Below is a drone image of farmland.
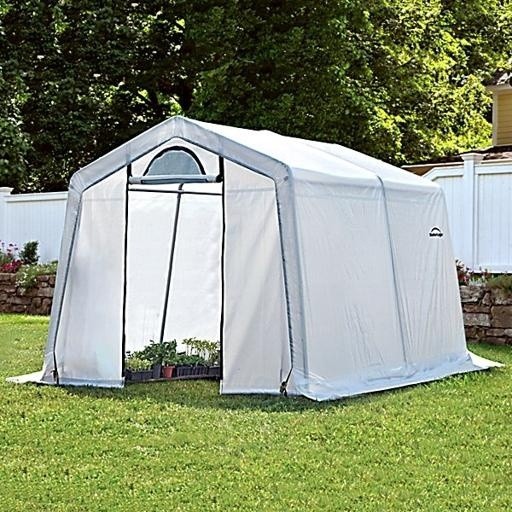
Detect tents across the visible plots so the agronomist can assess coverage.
[6,114,505,401]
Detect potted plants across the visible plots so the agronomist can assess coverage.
[121,339,224,383]
